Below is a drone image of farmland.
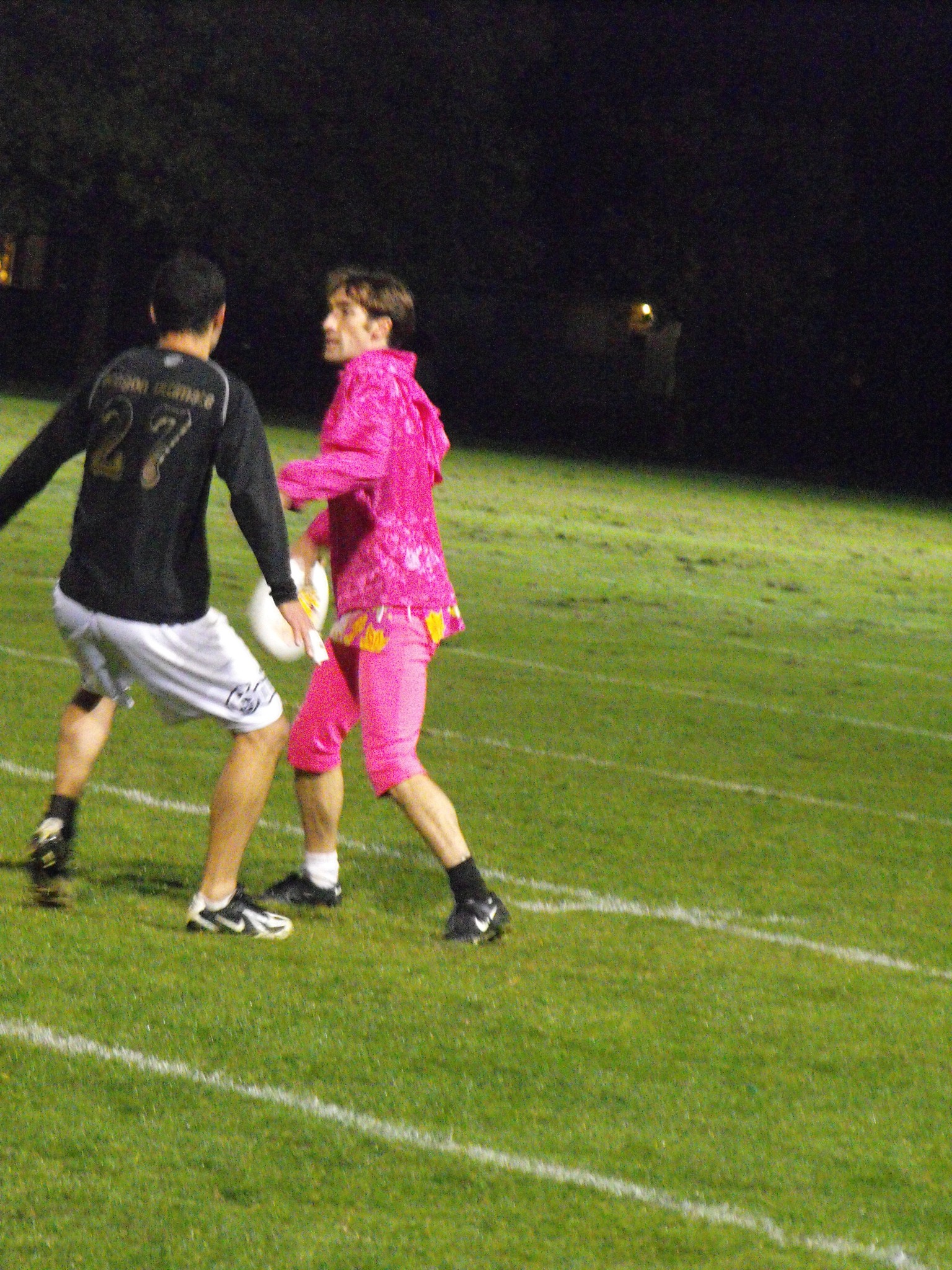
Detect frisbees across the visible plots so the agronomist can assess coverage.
[247,556,330,663]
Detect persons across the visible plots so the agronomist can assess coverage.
[250,269,511,946]
[0,254,329,941]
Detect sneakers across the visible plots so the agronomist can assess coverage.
[28,818,71,908]
[186,886,293,942]
[257,872,347,909]
[445,890,512,947]
